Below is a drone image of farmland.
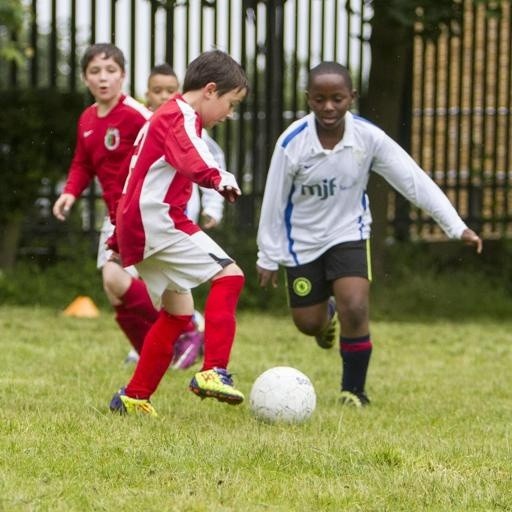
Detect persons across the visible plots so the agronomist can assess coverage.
[53,43,205,369]
[110,51,252,416]
[255,60,482,408]
[127,62,226,364]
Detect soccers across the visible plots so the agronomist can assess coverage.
[250,365,316,426]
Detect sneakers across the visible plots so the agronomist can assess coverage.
[316,295,338,349]
[109,385,161,420]
[337,390,369,408]
[188,366,245,406]
[169,309,206,371]
